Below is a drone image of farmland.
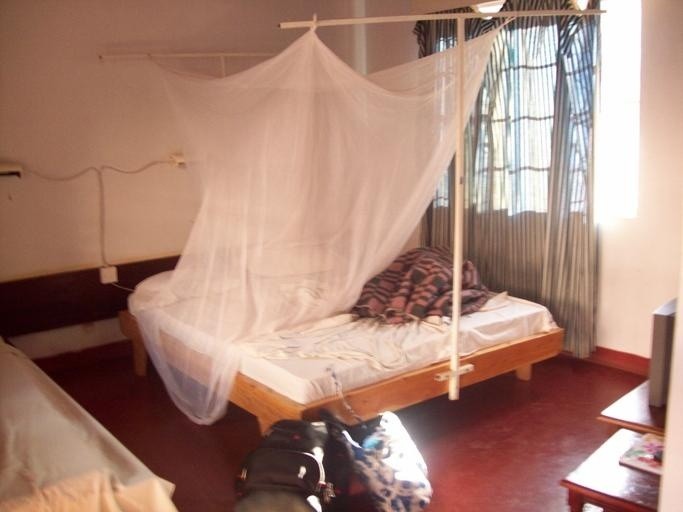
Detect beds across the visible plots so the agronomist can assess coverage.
[117,272,566,437]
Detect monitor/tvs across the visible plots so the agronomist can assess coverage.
[648,297,677,407]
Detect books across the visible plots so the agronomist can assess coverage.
[619,433,666,476]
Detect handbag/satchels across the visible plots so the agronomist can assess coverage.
[320,410,433,512]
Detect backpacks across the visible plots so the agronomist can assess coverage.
[233,419,349,512]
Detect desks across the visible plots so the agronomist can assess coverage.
[561,380,664,512]
